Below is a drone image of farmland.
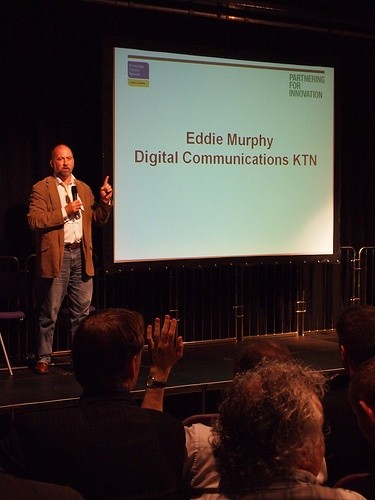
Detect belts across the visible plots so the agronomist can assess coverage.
[65,243,80,250]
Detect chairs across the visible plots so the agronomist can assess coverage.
[177,403,375,500]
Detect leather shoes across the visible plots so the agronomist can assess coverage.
[35,363,48,373]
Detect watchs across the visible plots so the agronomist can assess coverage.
[146,376,166,388]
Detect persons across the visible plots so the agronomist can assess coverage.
[28,144,112,375]
[317,305,374,500]
[187,360,365,500]
[2,308,185,500]
[141,314,327,489]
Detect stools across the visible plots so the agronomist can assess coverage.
[0,309,26,376]
[59,306,97,318]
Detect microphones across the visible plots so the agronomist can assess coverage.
[71,185,80,217]
